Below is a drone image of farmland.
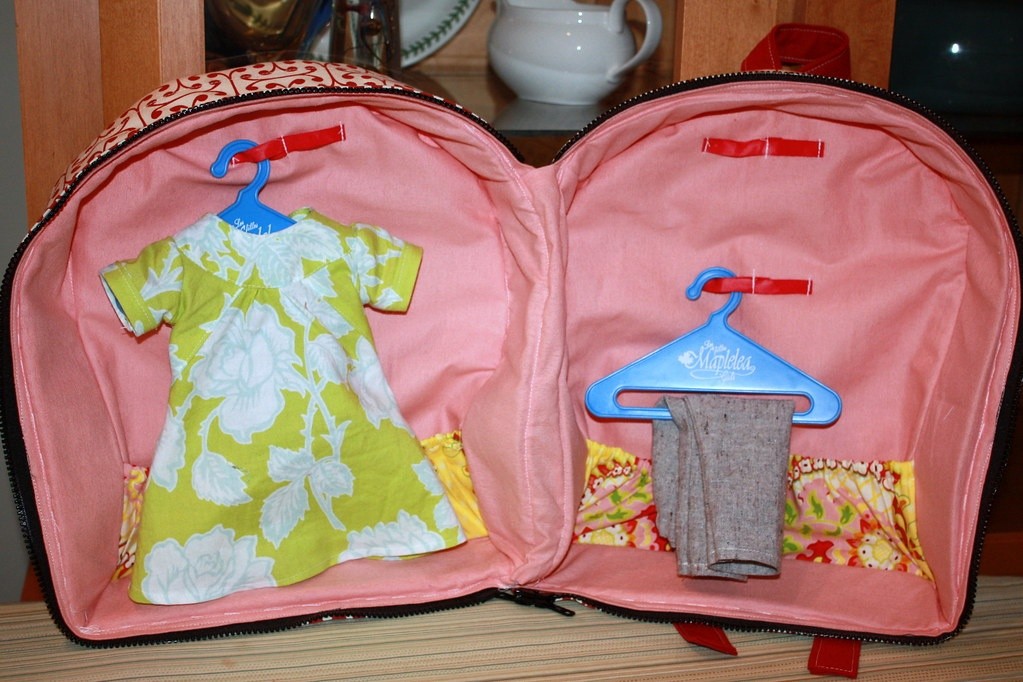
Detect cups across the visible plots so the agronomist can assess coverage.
[331,1,400,75]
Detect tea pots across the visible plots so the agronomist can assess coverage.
[485,2,665,107]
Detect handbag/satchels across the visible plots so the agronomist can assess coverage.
[1,62,1022,645]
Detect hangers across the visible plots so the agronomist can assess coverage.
[585,266,843,428]
[112,137,419,314]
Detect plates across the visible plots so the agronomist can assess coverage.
[307,1,478,69]
